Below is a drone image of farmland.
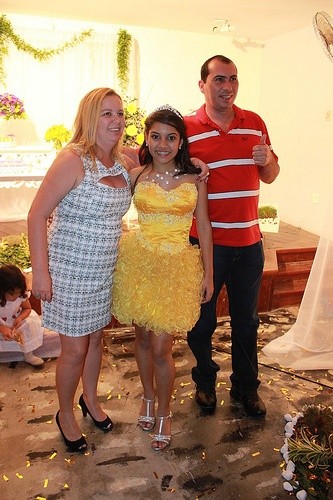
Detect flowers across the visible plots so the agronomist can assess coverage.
[119,94,148,151]
[0,89,29,127]
[1,234,31,269]
[43,122,74,151]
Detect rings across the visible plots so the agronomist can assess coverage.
[35,293,40,297]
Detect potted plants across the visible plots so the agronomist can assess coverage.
[256,204,281,235]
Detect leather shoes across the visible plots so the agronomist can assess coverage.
[229,384,266,416]
[194,383,216,409]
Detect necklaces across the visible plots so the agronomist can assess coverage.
[146,168,187,185]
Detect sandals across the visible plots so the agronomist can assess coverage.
[151,409,172,450]
[137,396,155,431]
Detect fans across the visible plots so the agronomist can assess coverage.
[311,9,333,65]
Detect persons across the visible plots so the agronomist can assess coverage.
[27,87,209,453]
[0,265,44,365]
[111,104,214,451]
[118,55,280,417]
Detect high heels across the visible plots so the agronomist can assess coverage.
[54,410,87,452]
[78,393,113,431]
